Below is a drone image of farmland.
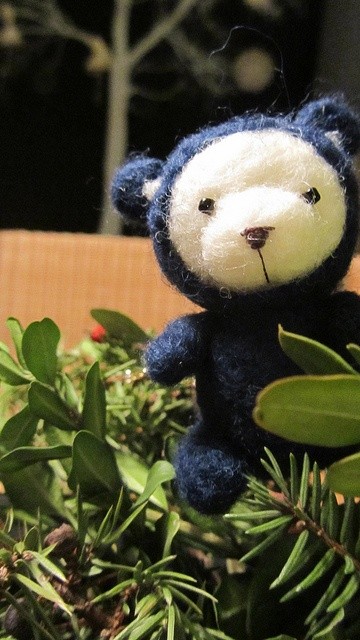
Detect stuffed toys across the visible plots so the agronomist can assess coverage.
[108,94,360,515]
